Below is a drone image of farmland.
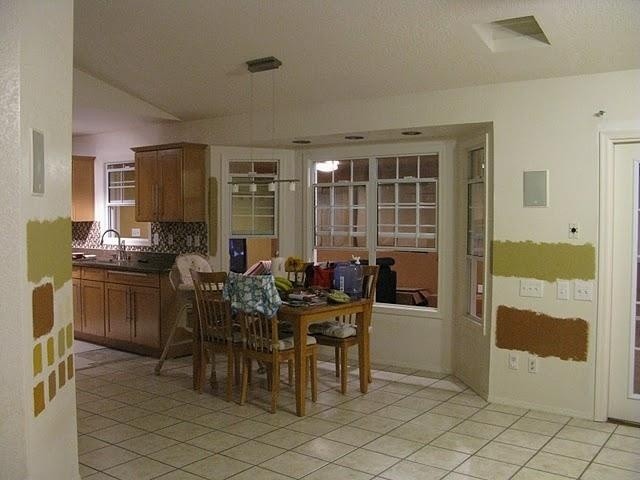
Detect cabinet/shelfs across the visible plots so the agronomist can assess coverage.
[129,142,208,224]
[72,265,161,358]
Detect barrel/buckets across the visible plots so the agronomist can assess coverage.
[333,261,364,301]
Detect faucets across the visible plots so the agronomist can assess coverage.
[100,229,125,260]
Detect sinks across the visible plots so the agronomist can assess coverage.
[97,259,116,265]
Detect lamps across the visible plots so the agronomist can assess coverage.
[227,56,300,193]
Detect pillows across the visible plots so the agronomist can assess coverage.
[241,260,273,275]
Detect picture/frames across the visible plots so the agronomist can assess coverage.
[30,127,46,196]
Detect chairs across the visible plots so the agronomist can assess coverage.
[153,251,381,414]
